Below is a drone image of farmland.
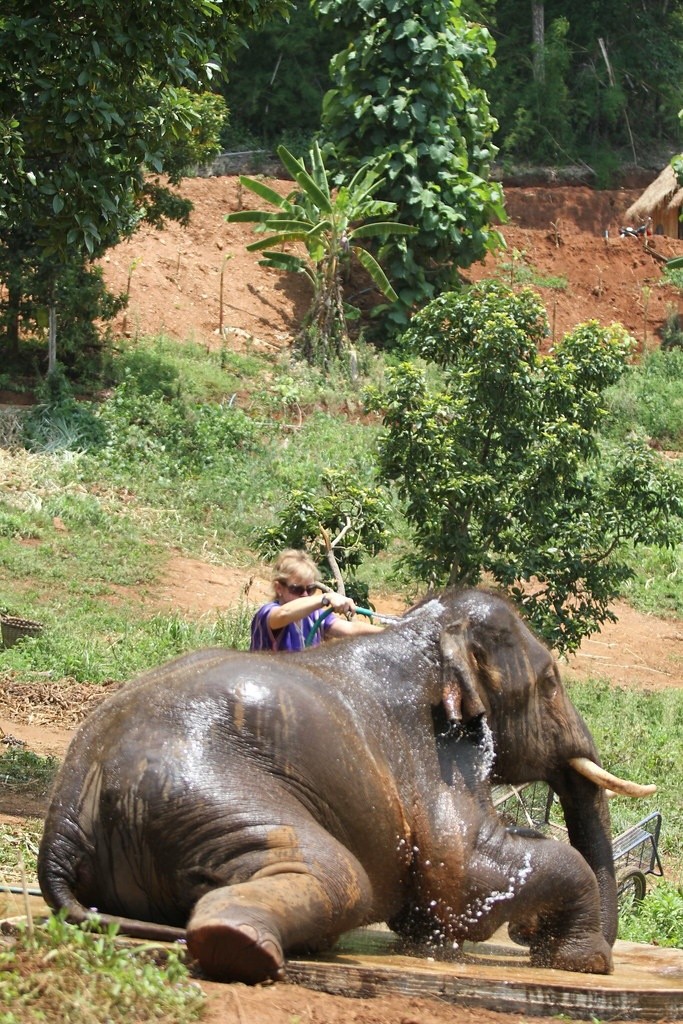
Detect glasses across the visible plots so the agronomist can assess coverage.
[277,579,316,596]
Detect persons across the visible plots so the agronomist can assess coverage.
[249,548,385,651]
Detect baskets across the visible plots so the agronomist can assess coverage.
[0,612,52,647]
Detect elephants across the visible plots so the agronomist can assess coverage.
[37,582,660,990]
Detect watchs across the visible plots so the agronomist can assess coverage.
[321,592,331,607]
